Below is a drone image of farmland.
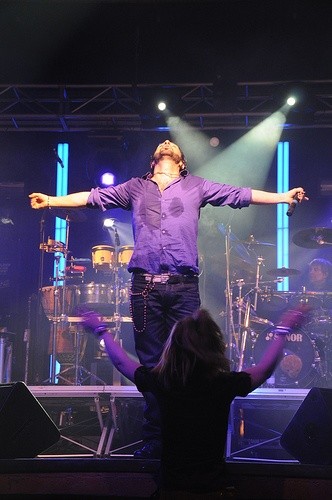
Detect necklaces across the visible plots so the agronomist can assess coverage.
[153,172,180,178]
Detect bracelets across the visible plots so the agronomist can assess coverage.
[46,196,51,209]
[271,325,294,335]
[94,324,108,335]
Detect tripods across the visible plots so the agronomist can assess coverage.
[43,324,105,386]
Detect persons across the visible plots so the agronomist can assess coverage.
[28,140,308,367]
[306,258,332,293]
[81,304,316,500]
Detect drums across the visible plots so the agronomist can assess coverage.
[306,308,332,327]
[39,285,74,354]
[90,245,115,269]
[117,246,134,267]
[54,325,88,386]
[76,283,114,308]
[252,325,324,388]
[119,279,131,307]
[231,269,272,312]
[248,294,287,328]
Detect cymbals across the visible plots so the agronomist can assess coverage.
[293,227,332,248]
[243,241,276,246]
[266,269,302,276]
[39,240,65,253]
[212,253,255,280]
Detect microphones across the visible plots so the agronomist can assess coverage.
[286,192,299,217]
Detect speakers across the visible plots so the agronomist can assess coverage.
[0,381,60,458]
[279,386,332,466]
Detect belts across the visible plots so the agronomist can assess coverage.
[132,274,198,285]
